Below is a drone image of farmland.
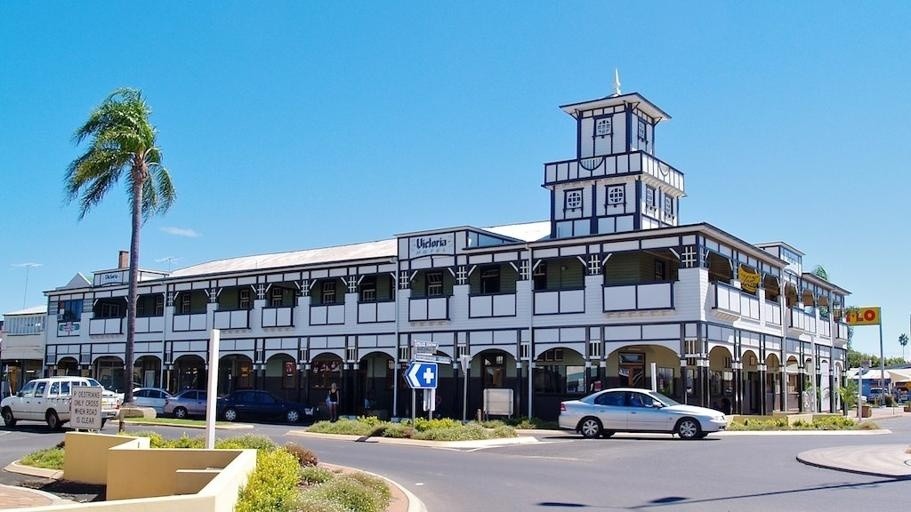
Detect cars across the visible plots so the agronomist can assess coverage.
[161,391,221,418]
[558,387,727,441]
[120,388,172,417]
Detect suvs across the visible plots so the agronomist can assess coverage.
[218,389,316,426]
[50,376,117,399]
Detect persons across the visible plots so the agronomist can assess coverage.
[326,389,331,407]
[327,382,339,423]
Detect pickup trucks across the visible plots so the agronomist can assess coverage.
[0,378,120,431]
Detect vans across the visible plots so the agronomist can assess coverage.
[867,387,888,402]
